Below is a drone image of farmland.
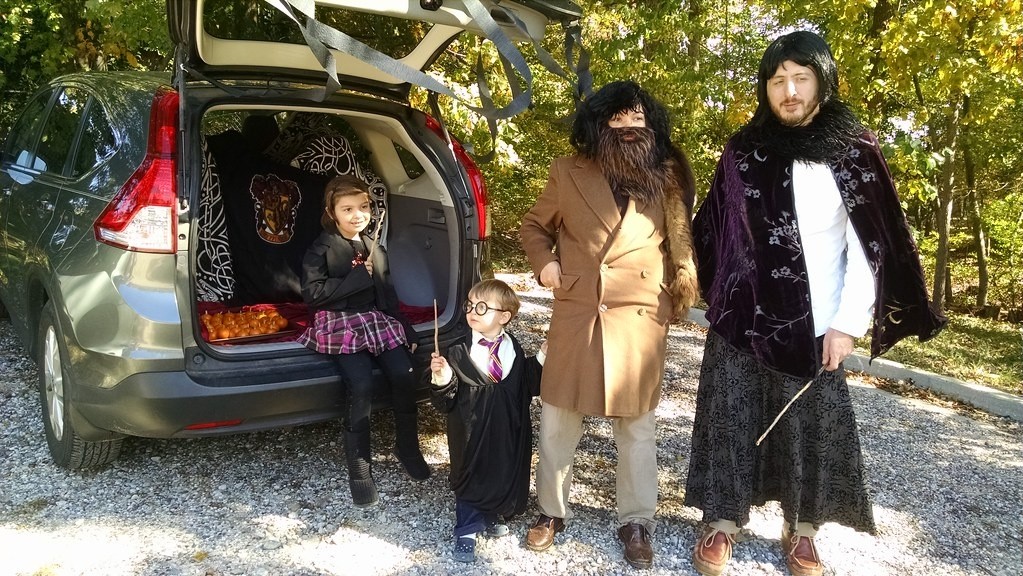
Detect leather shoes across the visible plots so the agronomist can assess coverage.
[618,521,653,568]
[525,512,566,551]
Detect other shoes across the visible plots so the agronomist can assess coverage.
[783,530,823,576]
[693,525,738,576]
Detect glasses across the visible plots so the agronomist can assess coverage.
[461,299,511,316]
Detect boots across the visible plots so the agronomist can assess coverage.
[342,430,379,507]
[391,412,431,482]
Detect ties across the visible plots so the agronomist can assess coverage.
[479,335,506,384]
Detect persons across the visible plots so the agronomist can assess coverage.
[431,281,547,563]
[683,31,943,576]
[302,174,432,506]
[520,79,697,570]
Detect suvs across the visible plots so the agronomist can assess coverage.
[0,0,588,472]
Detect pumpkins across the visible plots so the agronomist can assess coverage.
[202,308,288,339]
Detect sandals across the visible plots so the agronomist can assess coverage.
[483,519,510,536]
[453,535,477,563]
